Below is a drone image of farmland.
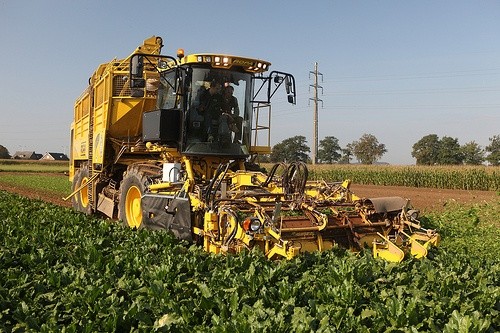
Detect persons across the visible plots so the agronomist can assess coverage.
[194,79,248,146]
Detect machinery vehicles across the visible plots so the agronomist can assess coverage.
[62,35,438,278]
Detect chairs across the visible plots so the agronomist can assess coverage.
[188,102,220,129]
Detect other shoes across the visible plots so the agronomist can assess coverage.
[233,139,241,144]
